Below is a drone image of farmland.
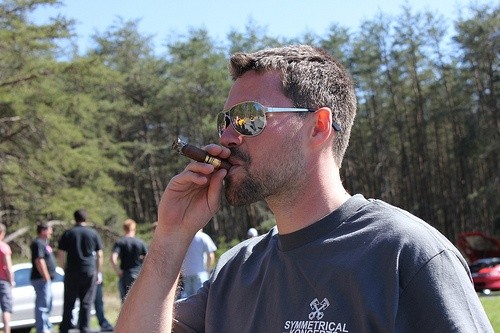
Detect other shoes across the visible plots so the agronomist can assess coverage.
[101,321,114,332]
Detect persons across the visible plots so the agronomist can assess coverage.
[0,224,15,333]
[113,45,494,333]
[249,113,257,132]
[246,227,259,239]
[111,218,147,304]
[177,224,217,299]
[30,221,55,333]
[60,208,113,333]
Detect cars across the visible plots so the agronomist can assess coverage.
[468,258,500,296]
[0,263,96,332]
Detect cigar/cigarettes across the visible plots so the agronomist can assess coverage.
[172,137,235,172]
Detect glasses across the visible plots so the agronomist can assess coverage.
[217,101,341,137]
